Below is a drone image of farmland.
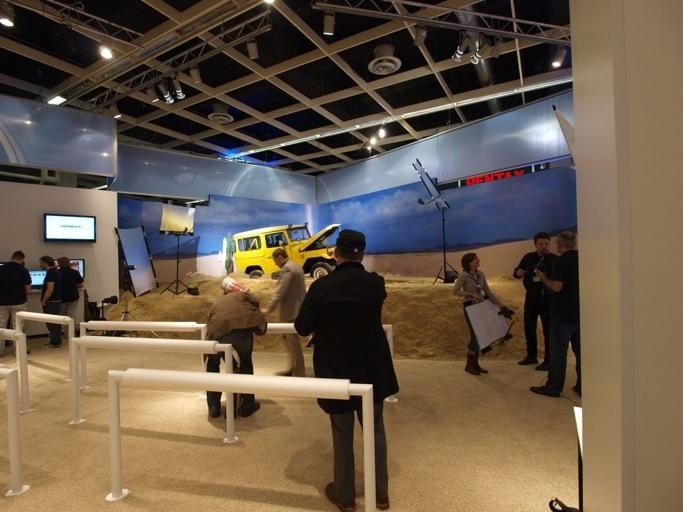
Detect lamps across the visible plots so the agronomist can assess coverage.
[320,8,571,70]
[106,35,260,121]
[358,121,388,153]
[0,0,116,63]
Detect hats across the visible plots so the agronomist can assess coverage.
[336,229,367,253]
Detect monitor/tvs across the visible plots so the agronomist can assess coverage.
[27,269,48,286]
[44,213,96,242]
[52,259,85,279]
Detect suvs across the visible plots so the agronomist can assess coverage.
[231,221,342,280]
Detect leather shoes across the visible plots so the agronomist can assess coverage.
[530,385,560,398]
[573,385,581,398]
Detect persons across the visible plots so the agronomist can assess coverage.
[262,248,307,377]
[530,230,581,398]
[0,251,32,357]
[40,256,62,350]
[57,257,86,339]
[202,290,267,418]
[453,253,508,375]
[294,229,399,512]
[513,232,559,370]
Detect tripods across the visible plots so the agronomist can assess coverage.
[159,235,190,295]
[111,311,161,338]
[91,301,110,336]
[432,210,462,286]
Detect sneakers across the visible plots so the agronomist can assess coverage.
[241,402,261,416]
[518,356,539,365]
[375,495,391,509]
[0,335,64,358]
[208,402,223,417]
[535,361,548,372]
[324,481,356,512]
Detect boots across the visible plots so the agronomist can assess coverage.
[464,352,489,375]
